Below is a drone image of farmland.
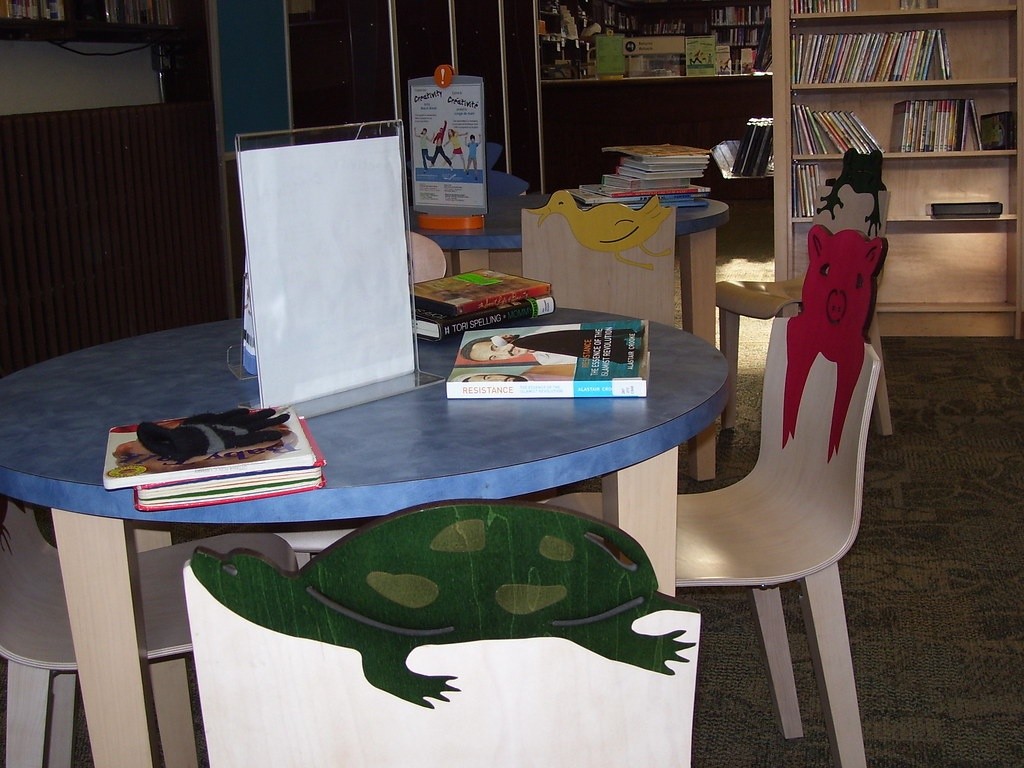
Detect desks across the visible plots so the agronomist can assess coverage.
[404,195,730,481]
[0,306,731,768]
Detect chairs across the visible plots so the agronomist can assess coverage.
[712,150,894,438]
[0,492,300,768]
[542,227,889,767]
[522,190,677,327]
[274,231,448,560]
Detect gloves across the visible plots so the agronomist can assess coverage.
[137,408,290,459]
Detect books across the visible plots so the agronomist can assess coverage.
[411,268,556,341]
[789,0,1017,220]
[565,144,711,212]
[0,0,65,20]
[596,0,772,75]
[103,405,326,512]
[446,320,656,399]
[730,117,773,178]
[105,0,173,24]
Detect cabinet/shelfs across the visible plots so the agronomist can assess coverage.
[769,0,1024,338]
